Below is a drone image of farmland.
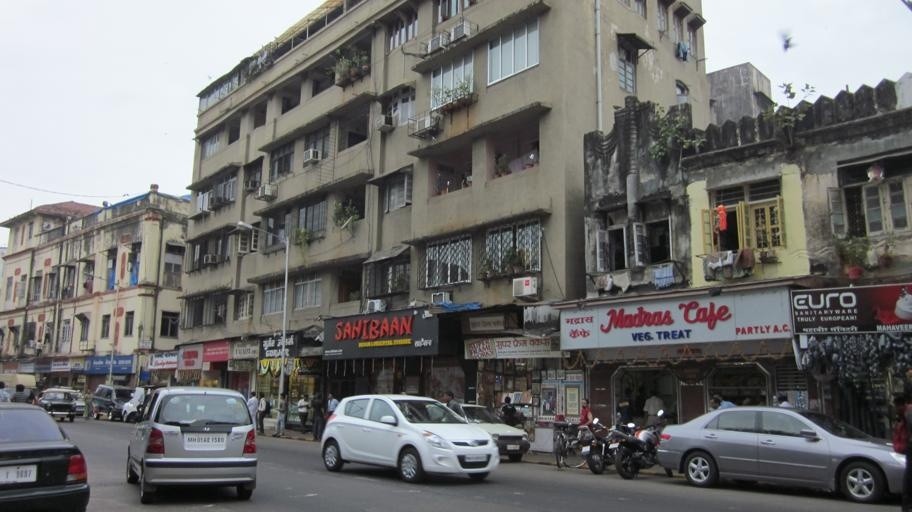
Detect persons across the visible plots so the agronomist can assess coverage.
[578,397,593,469]
[0,381,7,401]
[500,397,516,426]
[776,390,795,409]
[249,391,339,441]
[642,392,666,426]
[893,365,912,512]
[11,384,28,402]
[708,398,723,410]
[617,388,634,419]
[713,394,738,408]
[83,389,92,418]
[443,390,465,423]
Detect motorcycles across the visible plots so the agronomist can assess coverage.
[551,407,679,481]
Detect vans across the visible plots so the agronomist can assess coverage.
[126,387,258,504]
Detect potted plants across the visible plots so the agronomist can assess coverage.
[834,235,873,280]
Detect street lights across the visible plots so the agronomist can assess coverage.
[81,270,125,388]
[232,219,293,441]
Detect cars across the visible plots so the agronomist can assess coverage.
[319,394,500,487]
[0,401,91,512]
[654,404,907,505]
[427,400,530,462]
[2,381,164,428]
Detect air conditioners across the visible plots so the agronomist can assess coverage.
[259,181,277,196]
[303,149,320,164]
[203,252,217,265]
[511,275,538,300]
[366,299,385,313]
[415,115,437,135]
[451,22,473,42]
[427,29,449,52]
[430,291,452,306]
